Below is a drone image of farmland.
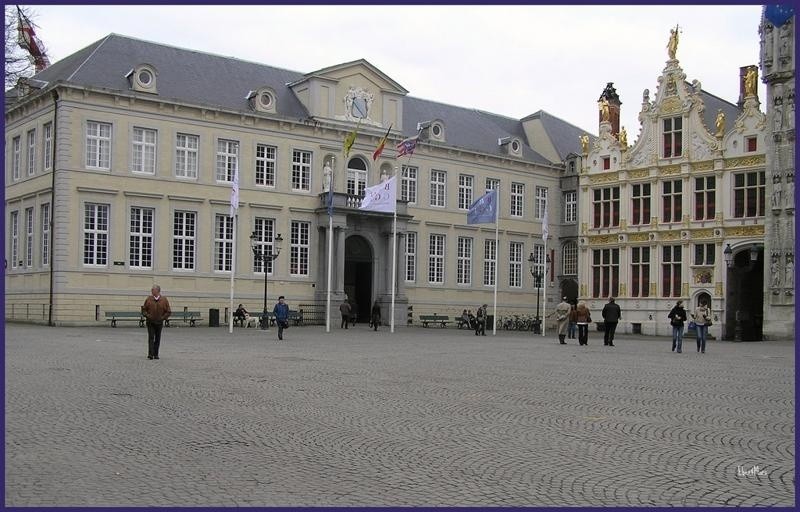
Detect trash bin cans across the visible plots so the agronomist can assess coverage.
[209,309,219,327]
[486,314,494,330]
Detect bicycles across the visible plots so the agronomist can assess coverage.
[496,314,537,331]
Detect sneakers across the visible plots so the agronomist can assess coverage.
[154,356,159,359]
[148,355,153,359]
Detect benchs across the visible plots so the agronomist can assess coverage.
[455,316,477,330]
[105,310,203,328]
[233,309,303,328]
[419,315,453,327]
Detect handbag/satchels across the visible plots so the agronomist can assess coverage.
[281,320,289,328]
[688,322,696,330]
[704,318,712,326]
[673,317,682,327]
[587,317,591,321]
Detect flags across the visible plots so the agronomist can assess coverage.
[466,188,497,225]
[322,119,418,216]
[229,160,240,218]
[541,200,550,241]
[16,5,52,75]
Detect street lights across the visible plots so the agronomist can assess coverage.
[723,243,760,342]
[527,252,551,334]
[249,232,282,328]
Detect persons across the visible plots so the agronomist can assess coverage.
[554,295,592,346]
[351,300,360,326]
[715,108,728,138]
[142,284,172,360]
[236,304,248,327]
[578,132,589,153]
[600,97,610,121]
[362,86,374,120]
[668,300,687,352]
[343,84,357,118]
[462,304,489,335]
[273,295,289,340]
[601,296,622,346]
[371,299,382,331]
[744,67,755,96]
[339,298,351,329]
[666,28,679,58]
[616,126,627,146]
[693,298,711,353]
